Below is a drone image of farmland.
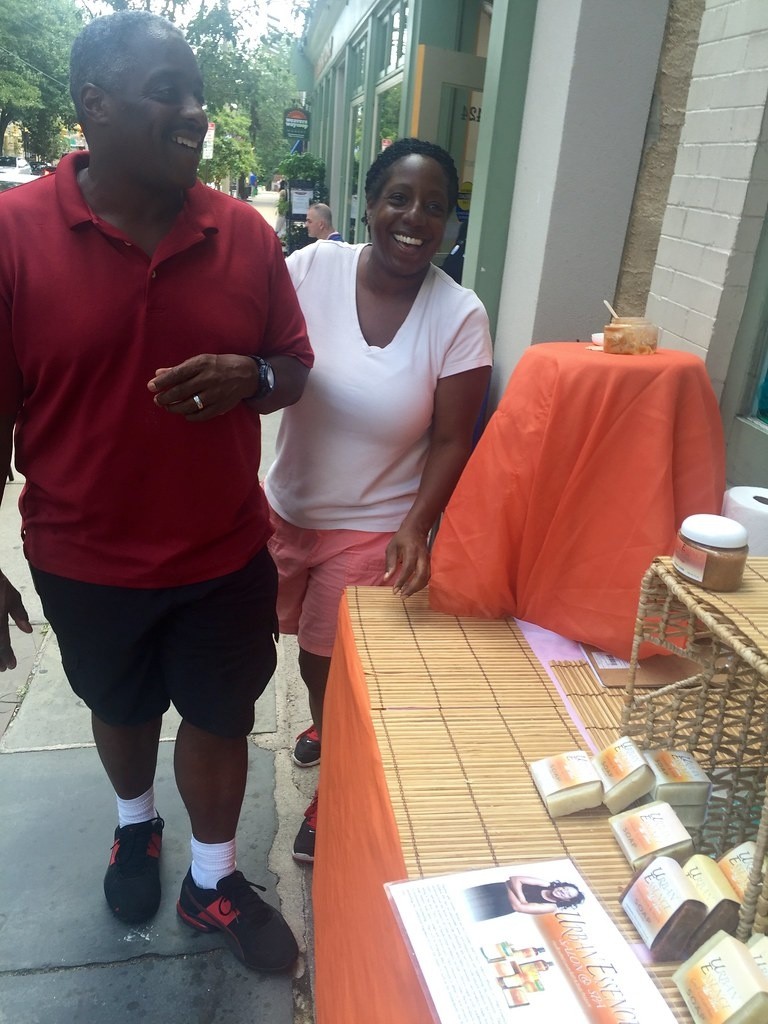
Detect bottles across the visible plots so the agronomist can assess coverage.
[603,317,658,355]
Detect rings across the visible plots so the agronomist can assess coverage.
[193,395,204,411]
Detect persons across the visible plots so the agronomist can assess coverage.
[262,136,496,862]
[0,9,315,976]
[304,203,346,242]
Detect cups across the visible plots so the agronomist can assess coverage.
[591,332,604,346]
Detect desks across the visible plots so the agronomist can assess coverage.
[310,586,768,1024]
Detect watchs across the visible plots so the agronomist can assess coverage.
[244,355,276,402]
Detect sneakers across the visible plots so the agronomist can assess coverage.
[177,865,299,971]
[292,723,321,766]
[104,808,165,924]
[292,788,318,861]
